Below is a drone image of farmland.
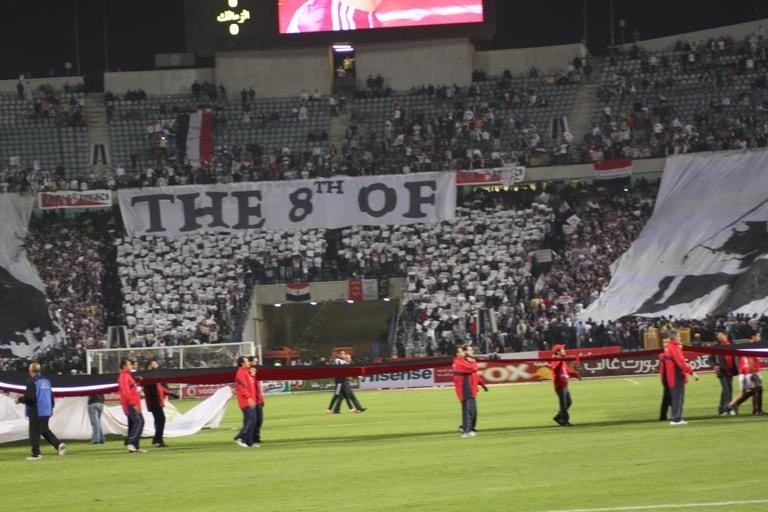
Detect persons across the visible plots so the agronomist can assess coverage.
[15,361,65,460]
[706,324,740,417]
[456,345,489,432]
[333,353,369,413]
[551,343,581,425]
[143,358,180,448]
[0,21,767,192]
[85,366,105,445]
[1,181,767,385]
[658,329,698,426]
[233,355,261,448]
[117,357,146,453]
[724,326,767,416]
[452,345,480,438]
[659,336,679,421]
[234,356,266,444]
[325,348,361,414]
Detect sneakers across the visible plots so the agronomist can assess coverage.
[127,444,136,452]
[235,437,262,448]
[27,455,42,461]
[458,425,478,438]
[327,409,340,417]
[754,410,768,416]
[350,407,367,413]
[554,415,574,427]
[659,416,669,421]
[669,419,687,425]
[137,448,147,453]
[58,444,67,457]
[718,404,739,416]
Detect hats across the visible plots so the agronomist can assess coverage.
[550,343,566,353]
